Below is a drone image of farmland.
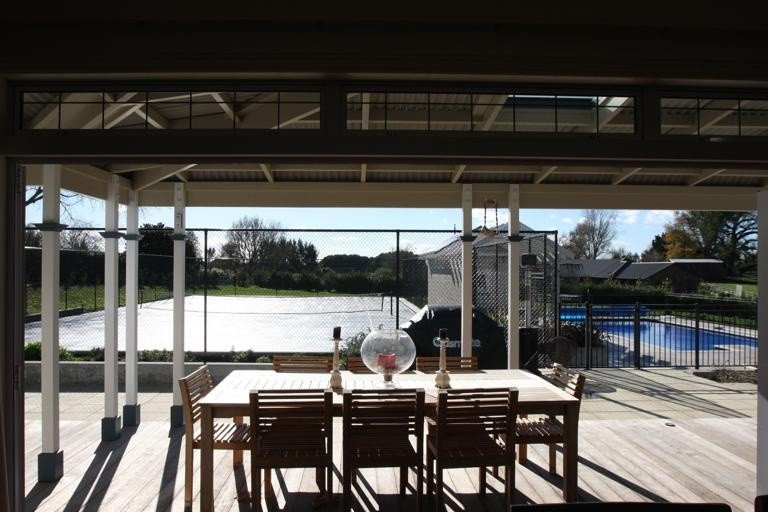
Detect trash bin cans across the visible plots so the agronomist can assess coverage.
[519,328,538,372]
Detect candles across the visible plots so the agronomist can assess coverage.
[437,325,449,339]
[331,325,342,339]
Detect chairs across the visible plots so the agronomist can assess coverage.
[178,353,586,512]
